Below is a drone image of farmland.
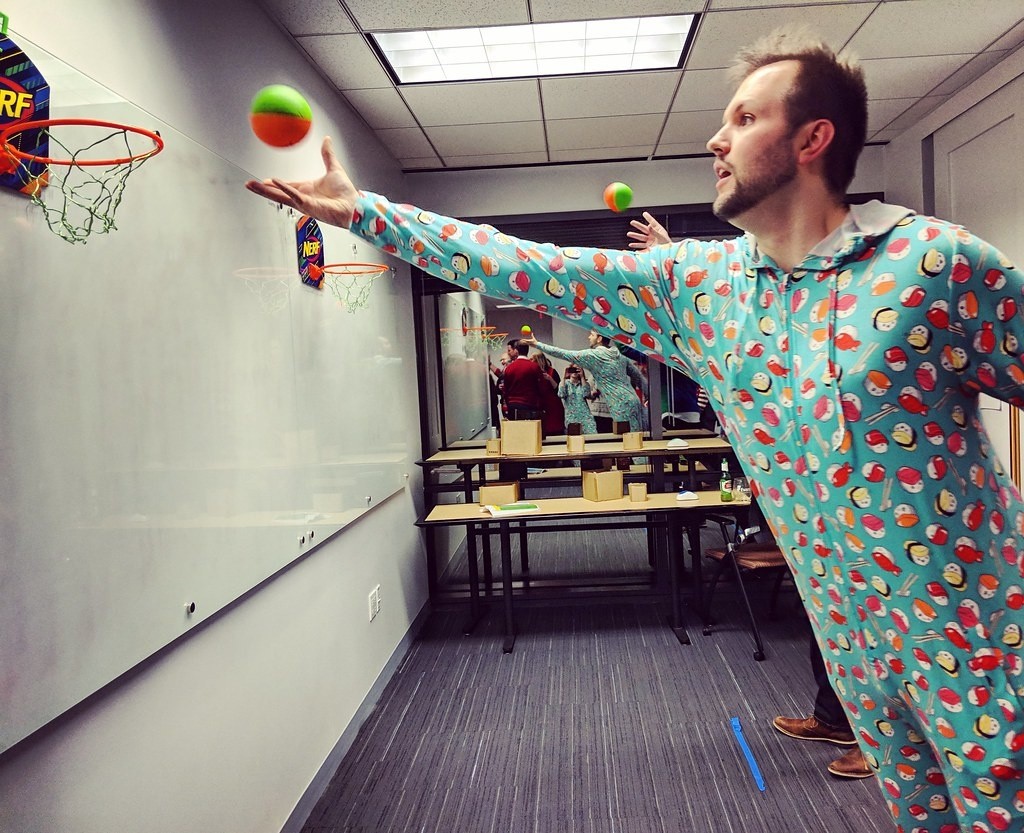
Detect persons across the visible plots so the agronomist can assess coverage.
[490,329,653,438]
[244,27,1024,833]
[624,210,875,783]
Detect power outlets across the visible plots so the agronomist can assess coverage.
[374,585,383,610]
[369,591,377,622]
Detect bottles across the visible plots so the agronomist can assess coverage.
[719,458,733,502]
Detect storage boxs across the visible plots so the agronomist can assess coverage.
[610,421,630,434]
[627,483,649,502]
[581,470,625,503]
[500,419,542,454]
[621,431,645,449]
[566,435,586,452]
[479,480,521,505]
[485,435,501,454]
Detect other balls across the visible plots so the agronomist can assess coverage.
[604,181,635,212]
[521,325,531,335]
[250,84,314,148]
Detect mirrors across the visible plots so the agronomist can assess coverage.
[416,275,736,485]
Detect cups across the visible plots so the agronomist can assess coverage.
[734,476,752,502]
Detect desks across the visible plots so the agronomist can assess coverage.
[415,487,753,654]
[425,437,733,596]
[448,428,720,450]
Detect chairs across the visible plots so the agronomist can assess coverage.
[698,499,791,661]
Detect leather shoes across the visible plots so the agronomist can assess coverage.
[829,749,873,779]
[775,713,861,746]
